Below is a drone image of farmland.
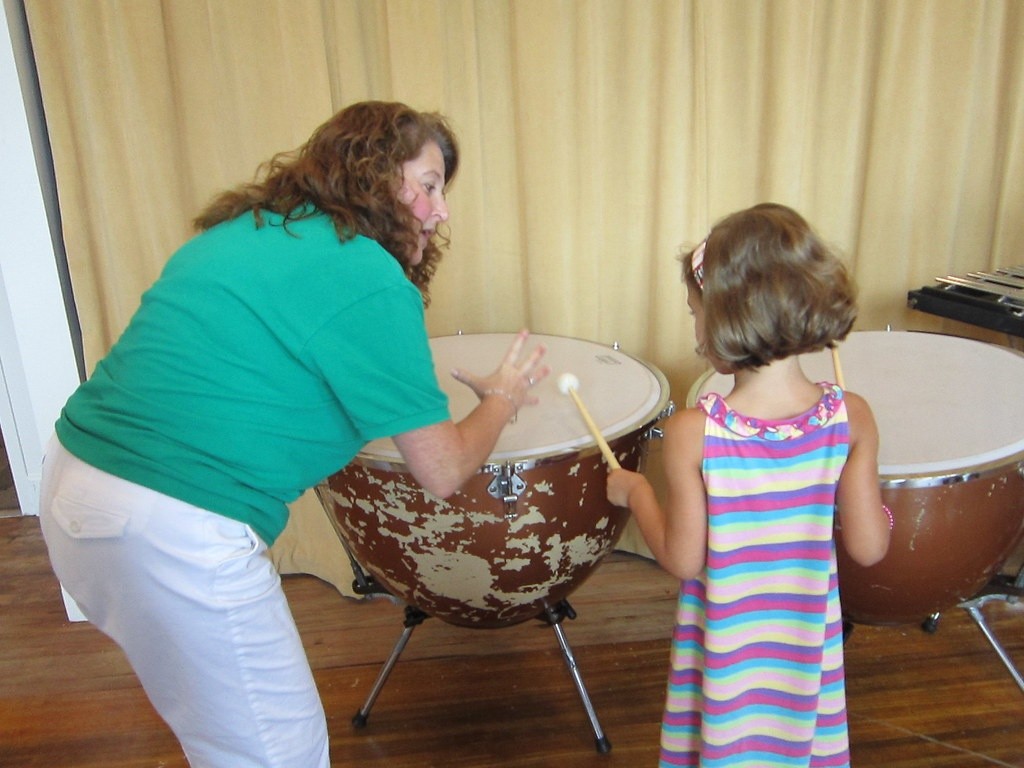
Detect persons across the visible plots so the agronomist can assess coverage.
[36,97,550,768]
[602,200,894,768]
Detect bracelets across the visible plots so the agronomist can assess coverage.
[881,504,893,531]
[480,388,519,425]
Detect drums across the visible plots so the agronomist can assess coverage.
[685,325,1024,629]
[322,330,675,629]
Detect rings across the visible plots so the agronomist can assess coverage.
[526,372,536,388]
